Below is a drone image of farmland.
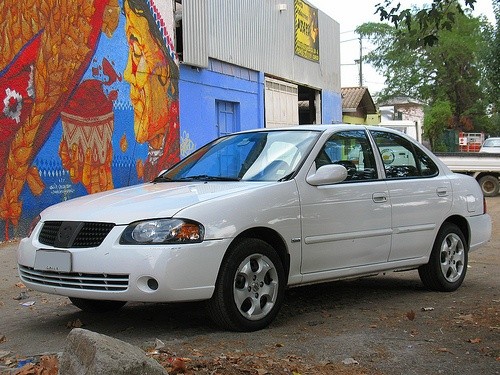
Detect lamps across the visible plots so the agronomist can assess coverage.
[280,3,287,14]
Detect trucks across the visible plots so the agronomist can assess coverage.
[359,120,500,198]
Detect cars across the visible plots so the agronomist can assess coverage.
[15,123,494,334]
[478,136,500,153]
